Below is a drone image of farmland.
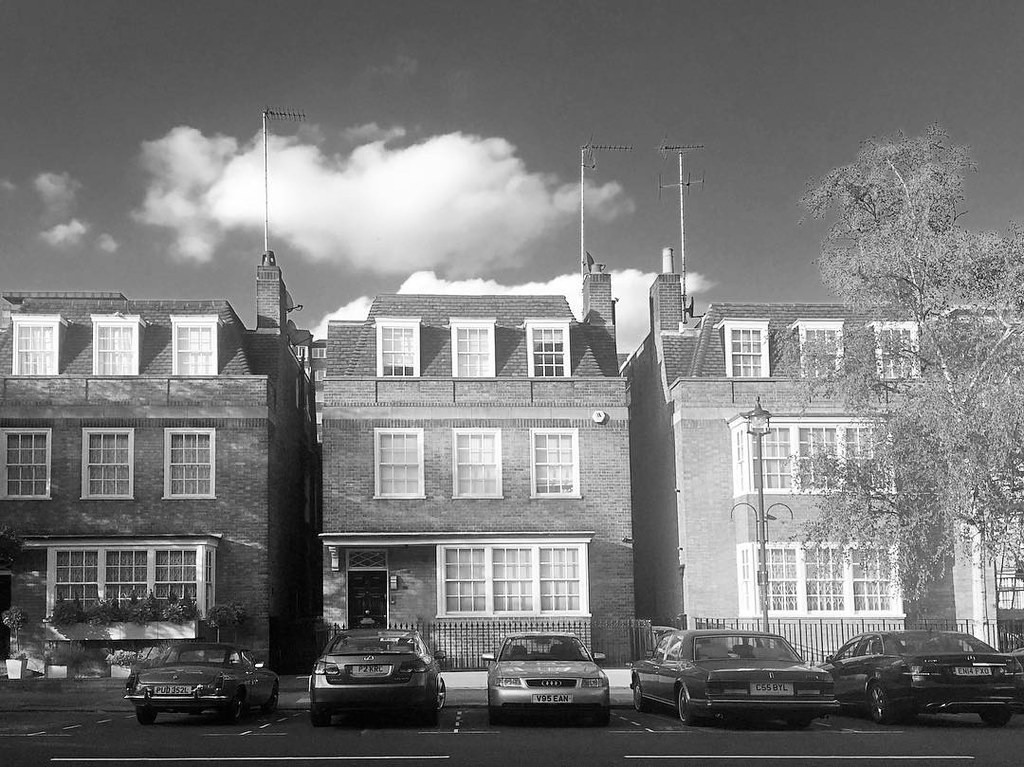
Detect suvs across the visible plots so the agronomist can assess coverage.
[311,628,448,727]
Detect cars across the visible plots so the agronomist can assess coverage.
[630,629,841,728]
[811,629,1024,727]
[123,644,279,723]
[481,632,611,728]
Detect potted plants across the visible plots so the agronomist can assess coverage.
[106,649,137,678]
[6,654,28,679]
[46,649,84,680]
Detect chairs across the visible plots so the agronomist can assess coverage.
[550,645,566,653]
[510,645,527,656]
[733,645,754,657]
[697,643,727,658]
[752,648,778,658]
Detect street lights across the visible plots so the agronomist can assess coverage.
[727,396,798,651]
